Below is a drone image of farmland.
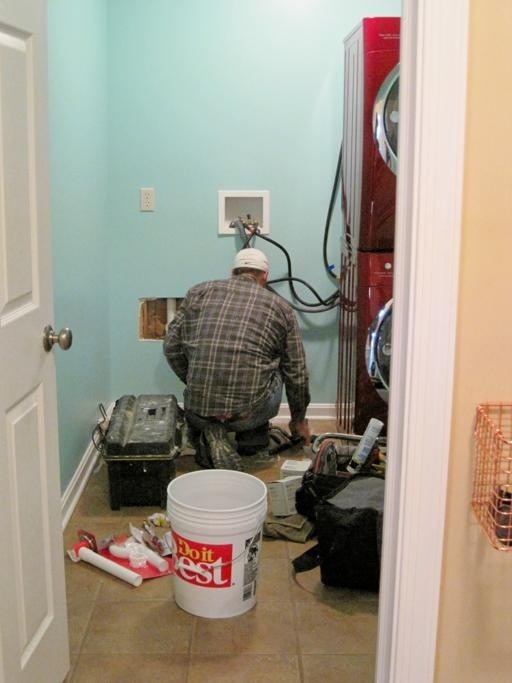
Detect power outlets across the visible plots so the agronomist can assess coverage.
[141,187,156,211]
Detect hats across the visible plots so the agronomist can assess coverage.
[230,247,269,274]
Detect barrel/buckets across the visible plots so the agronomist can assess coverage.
[166,470,267,619]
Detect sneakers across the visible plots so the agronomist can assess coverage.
[202,421,246,474]
[236,434,271,457]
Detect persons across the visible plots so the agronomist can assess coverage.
[161,245,313,470]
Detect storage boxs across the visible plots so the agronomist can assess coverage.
[104,392,181,512]
